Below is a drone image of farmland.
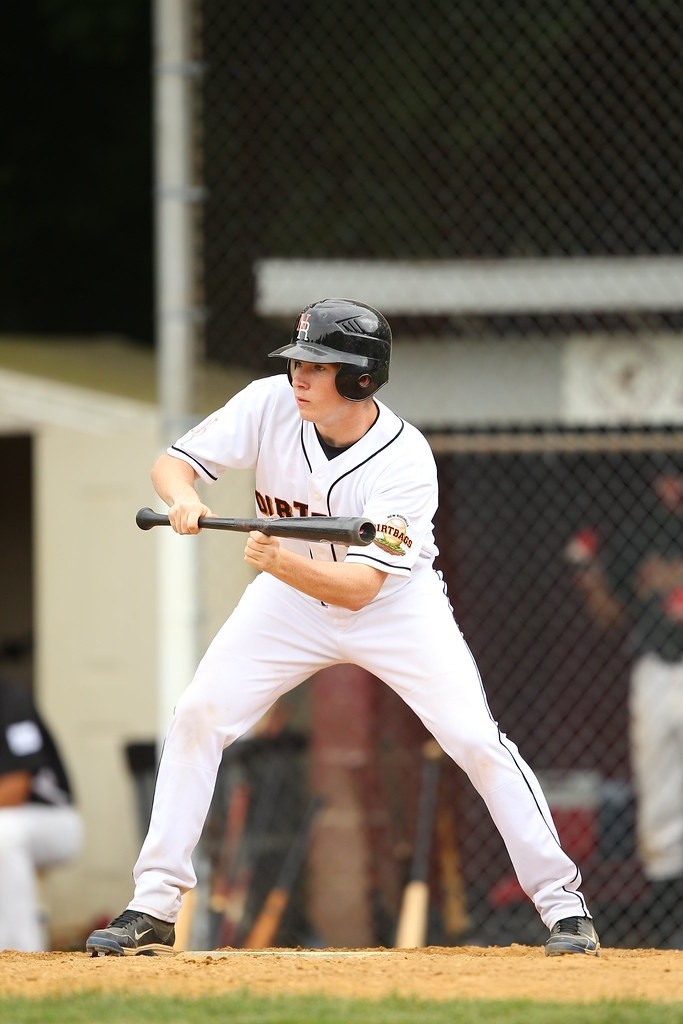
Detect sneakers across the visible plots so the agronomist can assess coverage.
[545,917,601,957]
[85,910,175,957]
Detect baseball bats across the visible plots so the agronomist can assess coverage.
[137,506,374,548]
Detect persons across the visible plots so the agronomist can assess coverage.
[88,299,600,957]
[222,687,313,946]
[567,467,683,884]
[0,680,81,951]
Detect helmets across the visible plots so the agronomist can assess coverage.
[267,297,391,402]
